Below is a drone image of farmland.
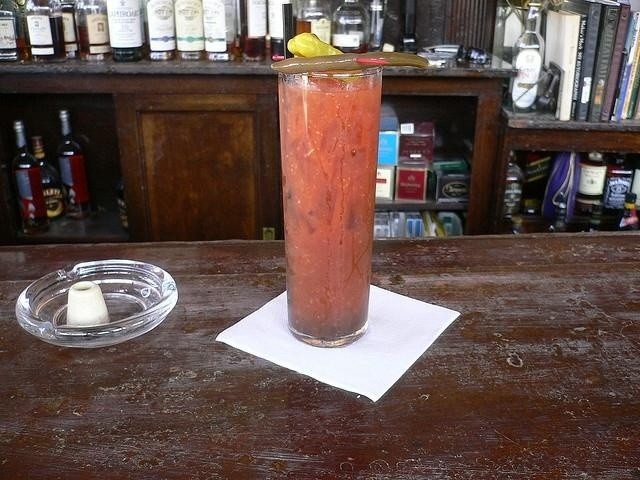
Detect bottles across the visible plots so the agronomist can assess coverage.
[505,146,640,230]
[30,135,66,220]
[294,0,332,52]
[332,1,370,54]
[116,177,129,233]
[54,109,92,219]
[504,3,545,112]
[10,119,50,234]
[0,0,294,64]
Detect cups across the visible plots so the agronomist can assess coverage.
[277,69,377,345]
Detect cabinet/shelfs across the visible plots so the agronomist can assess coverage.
[500,107,639,232]
[2,59,280,240]
[376,61,520,236]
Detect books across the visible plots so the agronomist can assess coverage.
[544,0,640,124]
